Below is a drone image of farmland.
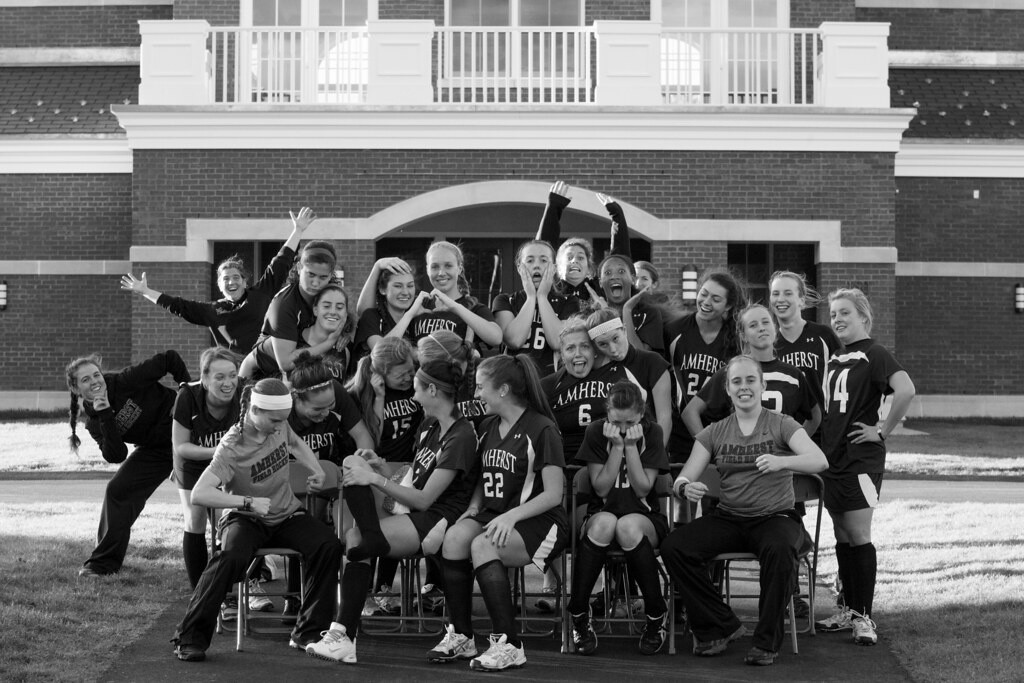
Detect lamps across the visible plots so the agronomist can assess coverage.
[682,264,698,307]
[333,265,344,290]
[1014,283,1024,312]
[0,280,8,310]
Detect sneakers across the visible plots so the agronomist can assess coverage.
[81,566,100,578]
[571,604,597,653]
[173,644,205,661]
[609,599,642,616]
[374,586,401,612]
[413,584,444,610]
[289,635,325,651]
[470,633,527,671]
[256,548,277,582]
[281,599,299,623]
[590,599,606,633]
[639,599,668,654]
[425,624,477,662]
[785,598,810,616]
[695,623,747,655]
[306,621,357,663]
[814,606,861,631]
[744,647,778,664]
[534,599,556,611]
[220,602,239,619]
[361,597,382,616]
[852,615,877,644]
[243,580,274,611]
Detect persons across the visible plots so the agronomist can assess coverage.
[659,355,829,665]
[815,287,915,646]
[426,354,569,672]
[306,360,478,664]
[565,378,670,655]
[121,180,842,620]
[170,378,343,661]
[66,350,192,575]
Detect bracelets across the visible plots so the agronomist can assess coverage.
[467,504,480,510]
[384,478,387,486]
[244,499,245,508]
[877,429,885,441]
[673,476,690,492]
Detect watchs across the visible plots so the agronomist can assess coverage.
[245,496,253,510]
[679,483,688,500]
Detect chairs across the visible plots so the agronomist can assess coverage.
[660,38,701,104]
[317,36,368,103]
[211,460,824,653]
